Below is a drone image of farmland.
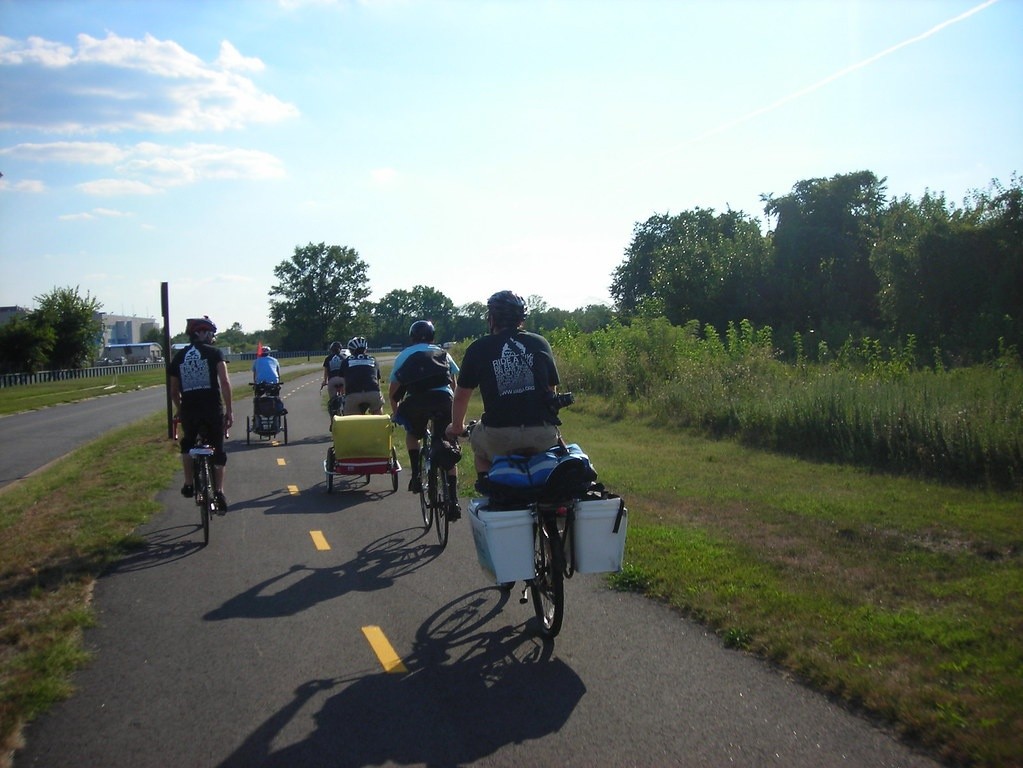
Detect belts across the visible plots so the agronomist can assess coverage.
[511,421,552,427]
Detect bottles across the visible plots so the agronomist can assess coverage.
[424,444,431,469]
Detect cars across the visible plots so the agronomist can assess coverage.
[95,356,128,366]
[135,357,166,364]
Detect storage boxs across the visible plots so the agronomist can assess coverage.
[570,496,628,574]
[466,498,536,584]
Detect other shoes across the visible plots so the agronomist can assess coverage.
[408,478,422,493]
[450,497,461,516]
[214,489,227,515]
[184,481,194,497]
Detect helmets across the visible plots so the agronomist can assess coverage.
[186,317,217,335]
[261,346,271,354]
[348,337,368,349]
[488,291,528,321]
[331,342,342,350]
[409,320,435,342]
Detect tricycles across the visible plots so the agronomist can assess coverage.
[246,382,288,445]
[324,380,403,493]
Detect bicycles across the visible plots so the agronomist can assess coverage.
[464,418,565,638]
[320,382,346,432]
[413,413,461,548]
[172,414,229,545]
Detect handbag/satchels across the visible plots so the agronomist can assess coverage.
[395,350,452,393]
[483,442,597,489]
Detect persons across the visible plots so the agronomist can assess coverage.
[252,346,280,395]
[339,336,386,416]
[340,345,351,359]
[323,341,345,431]
[168,316,233,515]
[444,290,562,495]
[387,320,461,522]
